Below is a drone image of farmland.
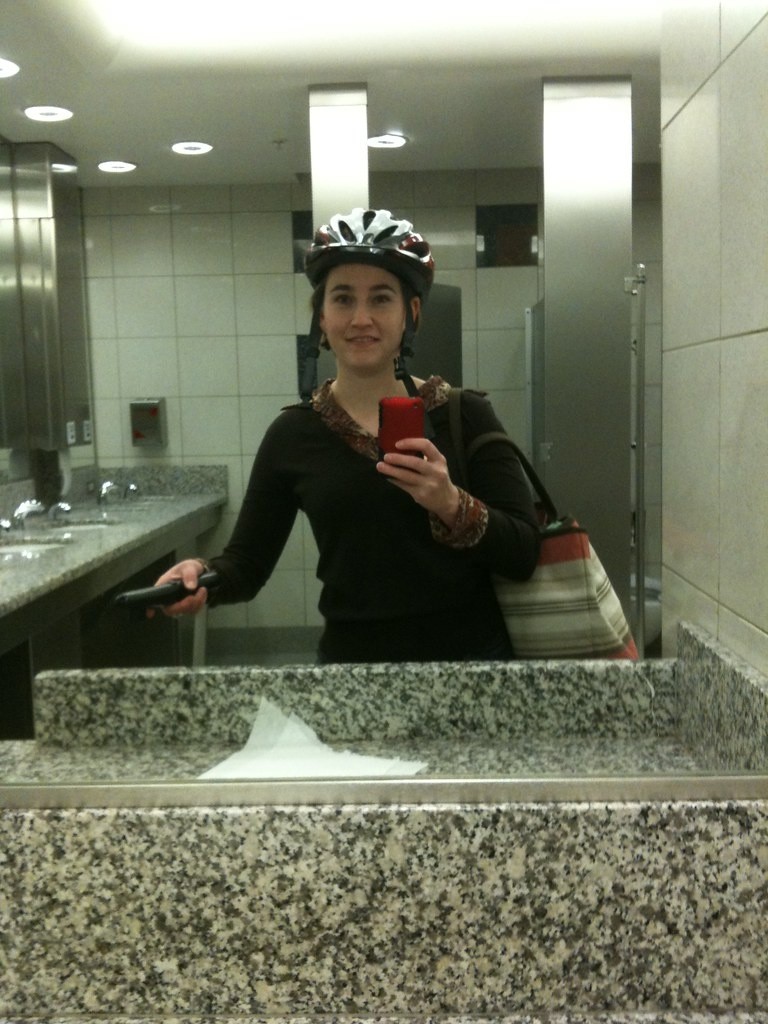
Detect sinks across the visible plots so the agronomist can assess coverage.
[0,533,82,553]
[32,518,123,533]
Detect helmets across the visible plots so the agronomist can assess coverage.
[304,208,434,303]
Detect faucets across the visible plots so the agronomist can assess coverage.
[47,502,72,520]
[0,518,12,532]
[96,480,140,519]
[13,499,45,529]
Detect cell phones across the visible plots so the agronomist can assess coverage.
[379,397,425,478]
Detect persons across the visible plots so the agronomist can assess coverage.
[146,210,545,665]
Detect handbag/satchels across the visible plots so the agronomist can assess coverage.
[448,387,639,660]
